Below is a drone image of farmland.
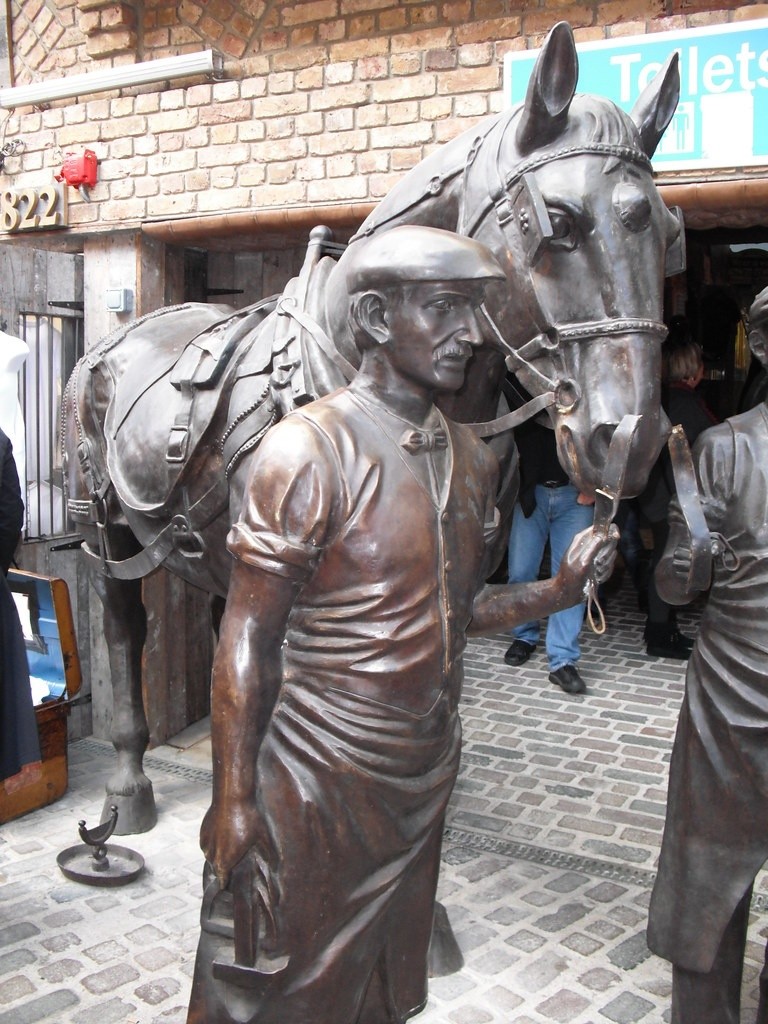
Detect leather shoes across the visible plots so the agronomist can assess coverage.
[504,639,536,665]
[549,666,585,693]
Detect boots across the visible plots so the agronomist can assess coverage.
[645,623,692,659]
[674,628,696,647]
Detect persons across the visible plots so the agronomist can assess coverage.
[0,428,25,580]
[501,345,706,694]
[647,288,767,1024]
[185,222,619,1024]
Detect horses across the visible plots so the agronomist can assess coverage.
[57,20,682,837]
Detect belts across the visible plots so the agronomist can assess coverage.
[541,482,567,487]
